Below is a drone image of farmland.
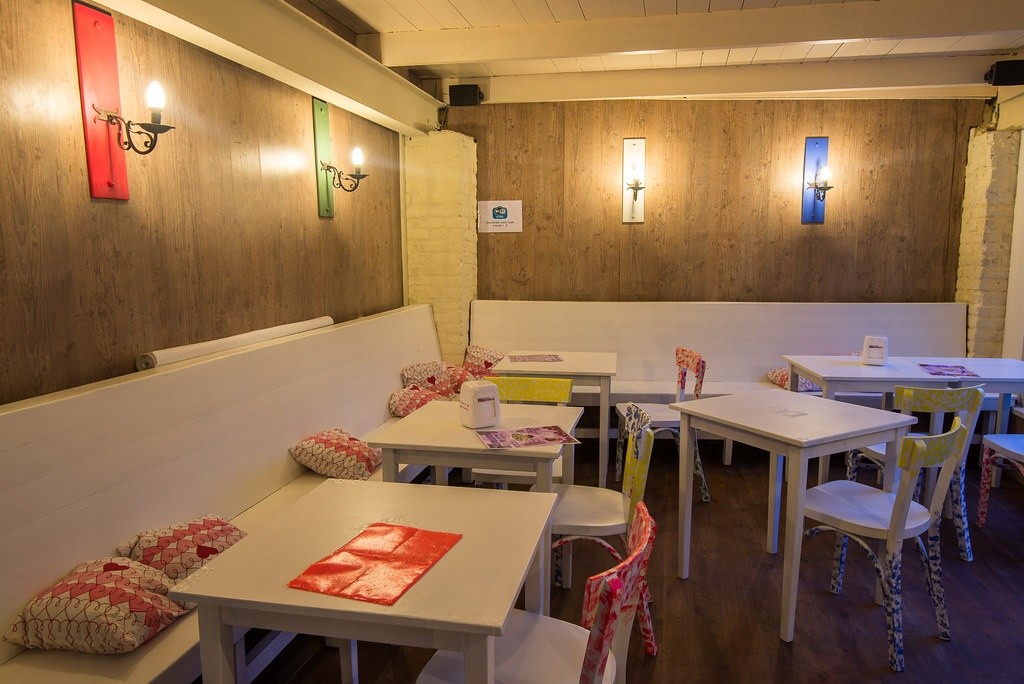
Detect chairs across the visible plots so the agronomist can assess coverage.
[472,375,577,490]
[846,383,985,564]
[952,392,1019,470]
[802,416,968,671]
[529,398,660,654]
[613,346,714,502]
[977,433,1024,529]
[415,498,659,684]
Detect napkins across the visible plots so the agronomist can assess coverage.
[285,521,463,608]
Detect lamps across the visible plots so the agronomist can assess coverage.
[72,0,177,203]
[622,137,646,225]
[312,96,370,219]
[801,136,834,226]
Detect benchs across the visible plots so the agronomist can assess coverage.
[470,298,969,466]
[0,303,445,684]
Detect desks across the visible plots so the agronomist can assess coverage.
[780,354,1024,486]
[492,350,620,489]
[668,388,917,643]
[365,400,584,617]
[163,476,558,684]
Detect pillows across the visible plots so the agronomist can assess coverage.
[388,383,450,419]
[289,426,383,481]
[1,554,198,657]
[463,345,505,380]
[117,512,249,582]
[447,363,477,394]
[769,366,823,393]
[401,360,457,402]
